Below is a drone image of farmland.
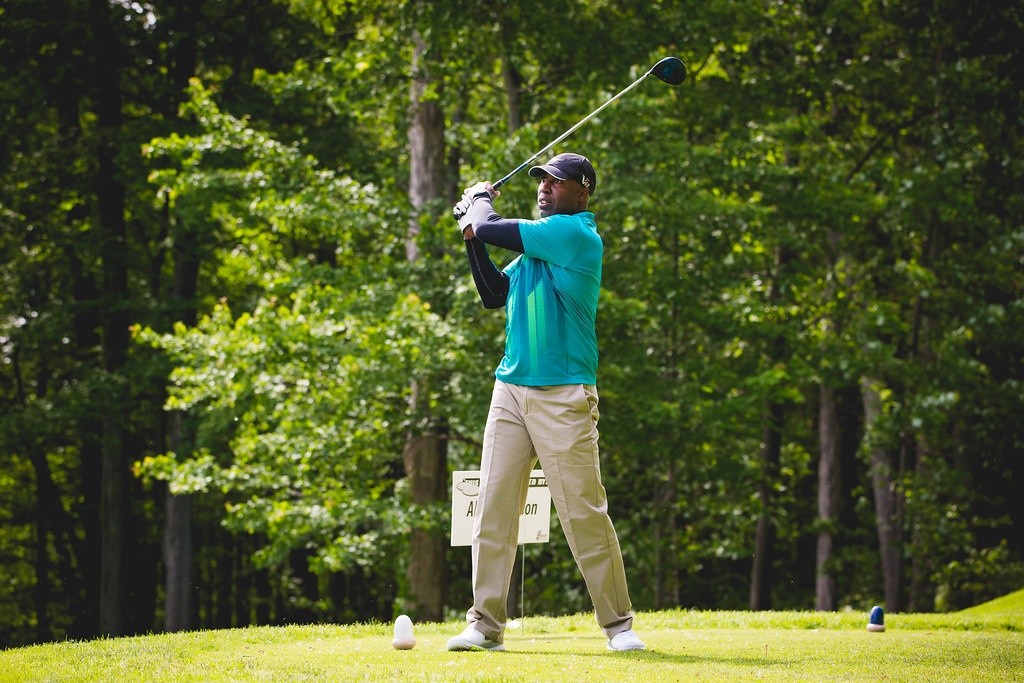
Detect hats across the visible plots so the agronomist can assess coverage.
[528,153,596,196]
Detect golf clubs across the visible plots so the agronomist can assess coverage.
[453,57,686,220]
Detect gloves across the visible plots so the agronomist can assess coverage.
[453,181,502,233]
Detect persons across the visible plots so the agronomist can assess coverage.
[445,151,648,653]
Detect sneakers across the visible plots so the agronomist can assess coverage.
[448,625,507,651]
[607,630,644,652]
